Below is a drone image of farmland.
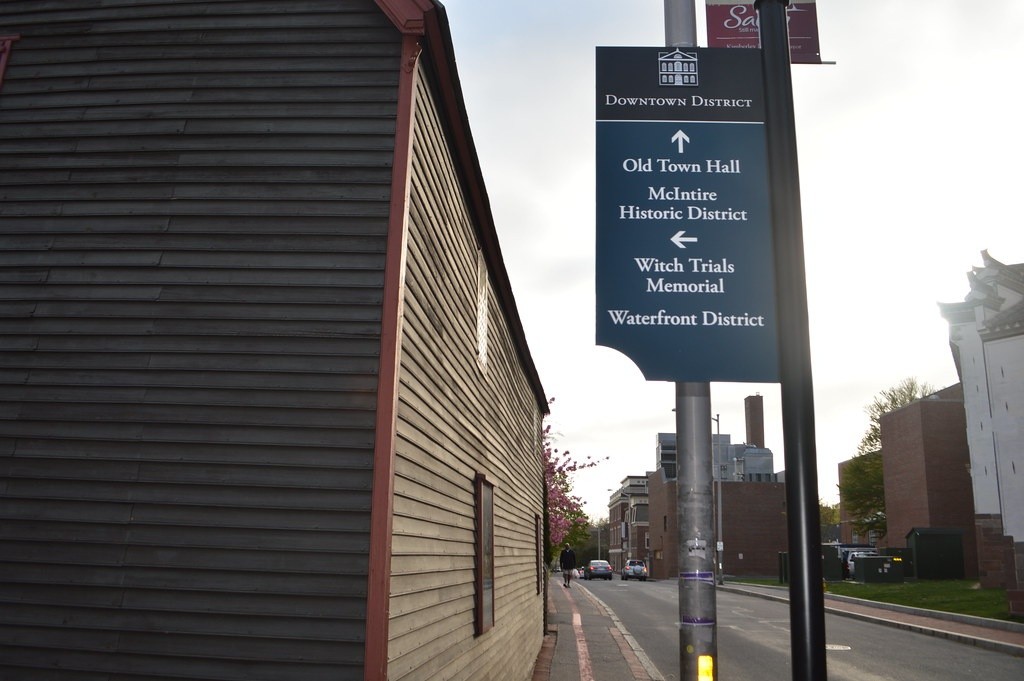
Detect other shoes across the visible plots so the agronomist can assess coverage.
[568,585,571,588]
[563,584,567,587]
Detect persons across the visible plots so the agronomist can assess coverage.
[560,542,576,588]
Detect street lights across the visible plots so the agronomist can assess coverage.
[671,408,724,585]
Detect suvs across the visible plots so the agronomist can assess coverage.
[621,560,647,581]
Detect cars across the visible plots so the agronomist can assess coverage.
[848,551,880,578]
[573,560,613,580]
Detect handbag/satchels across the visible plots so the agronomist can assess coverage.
[572,568,580,579]
